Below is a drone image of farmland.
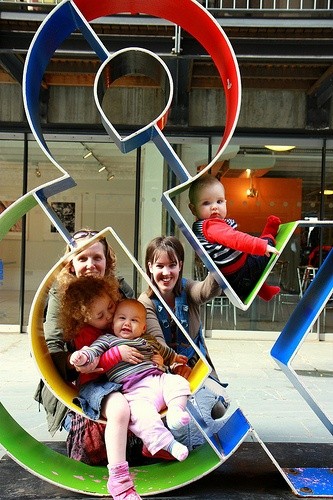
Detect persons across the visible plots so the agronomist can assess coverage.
[188,174,281,302]
[71,299,192,462]
[34,228,135,436]
[138,235,227,420]
[59,273,143,500]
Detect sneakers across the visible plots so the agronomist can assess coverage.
[107,461,142,500]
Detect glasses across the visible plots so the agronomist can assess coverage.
[72,230,107,243]
